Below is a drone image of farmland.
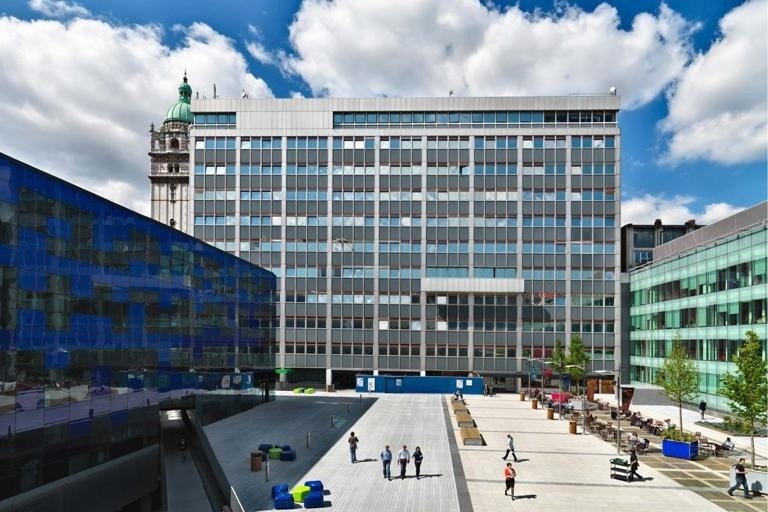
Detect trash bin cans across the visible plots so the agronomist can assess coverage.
[520,392,525,401]
[251,451,262,472]
[569,421,576,434]
[547,408,554,419]
[328,385,332,392]
[532,399,538,409]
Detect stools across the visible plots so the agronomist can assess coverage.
[258,443,296,461]
[270,481,323,509]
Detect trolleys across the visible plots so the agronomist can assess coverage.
[610,458,634,482]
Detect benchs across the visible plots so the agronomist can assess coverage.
[450,393,484,444]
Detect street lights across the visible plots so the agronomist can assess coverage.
[515,353,623,456]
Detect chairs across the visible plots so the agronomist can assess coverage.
[699,436,735,458]
